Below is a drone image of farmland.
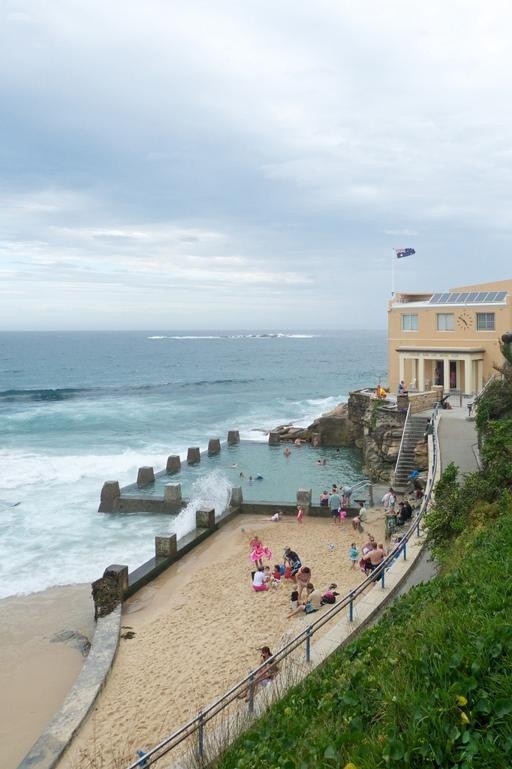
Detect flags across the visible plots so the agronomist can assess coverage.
[395,248,416,260]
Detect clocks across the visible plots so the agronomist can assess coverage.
[456,311,474,330]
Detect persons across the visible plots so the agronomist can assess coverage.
[375,385,386,399]
[451,368,456,388]
[238,646,275,702]
[434,367,440,383]
[238,438,420,620]
[398,381,403,394]
